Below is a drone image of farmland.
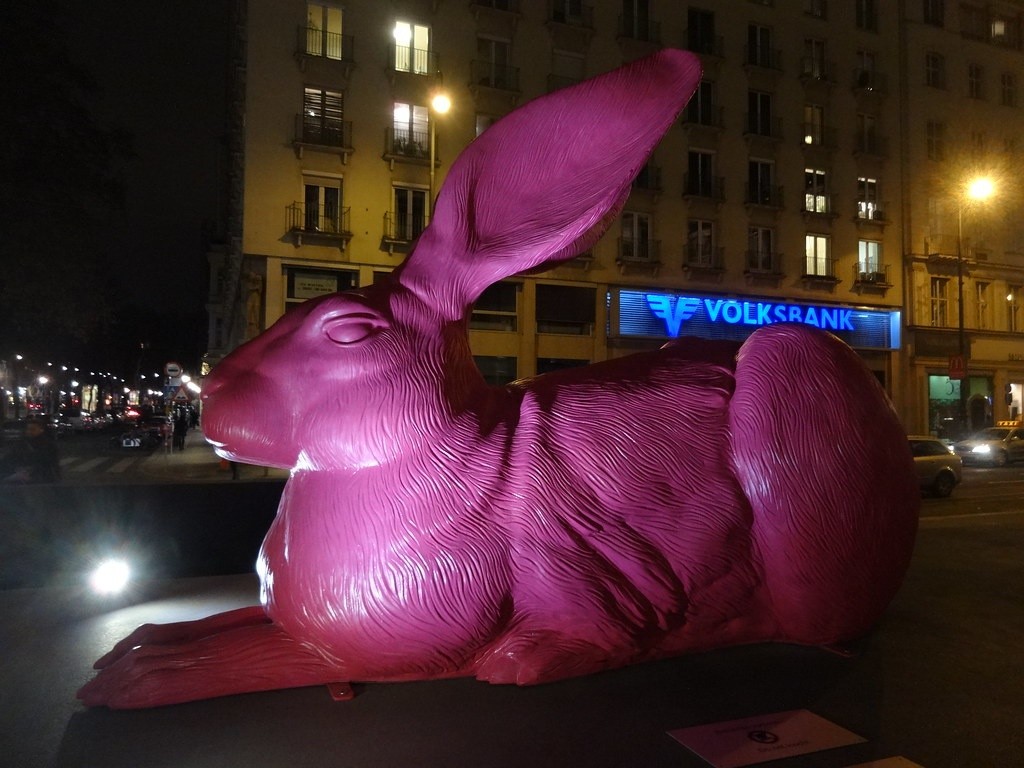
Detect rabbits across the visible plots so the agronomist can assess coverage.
[73,47,916,711]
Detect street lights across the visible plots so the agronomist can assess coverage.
[427,89,453,221]
[10,354,23,418]
[958,175,1000,418]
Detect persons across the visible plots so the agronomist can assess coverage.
[190,410,199,430]
[174,415,188,451]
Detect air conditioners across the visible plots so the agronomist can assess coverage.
[866,272,886,285]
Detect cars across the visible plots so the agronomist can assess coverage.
[48,406,145,437]
[908,435,963,497]
[947,420,1024,467]
